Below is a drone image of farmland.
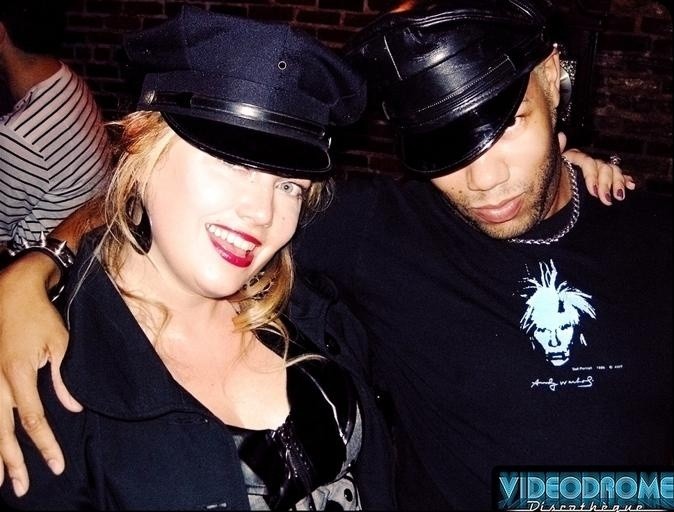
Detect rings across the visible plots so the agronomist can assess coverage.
[602,154,625,171]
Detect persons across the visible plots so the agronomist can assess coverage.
[519,257,597,369]
[0,0,113,253]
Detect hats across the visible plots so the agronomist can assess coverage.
[118,4,366,182]
[341,1,563,180]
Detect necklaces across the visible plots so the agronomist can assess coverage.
[505,153,581,246]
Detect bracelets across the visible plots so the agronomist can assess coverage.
[0,230,75,305]
